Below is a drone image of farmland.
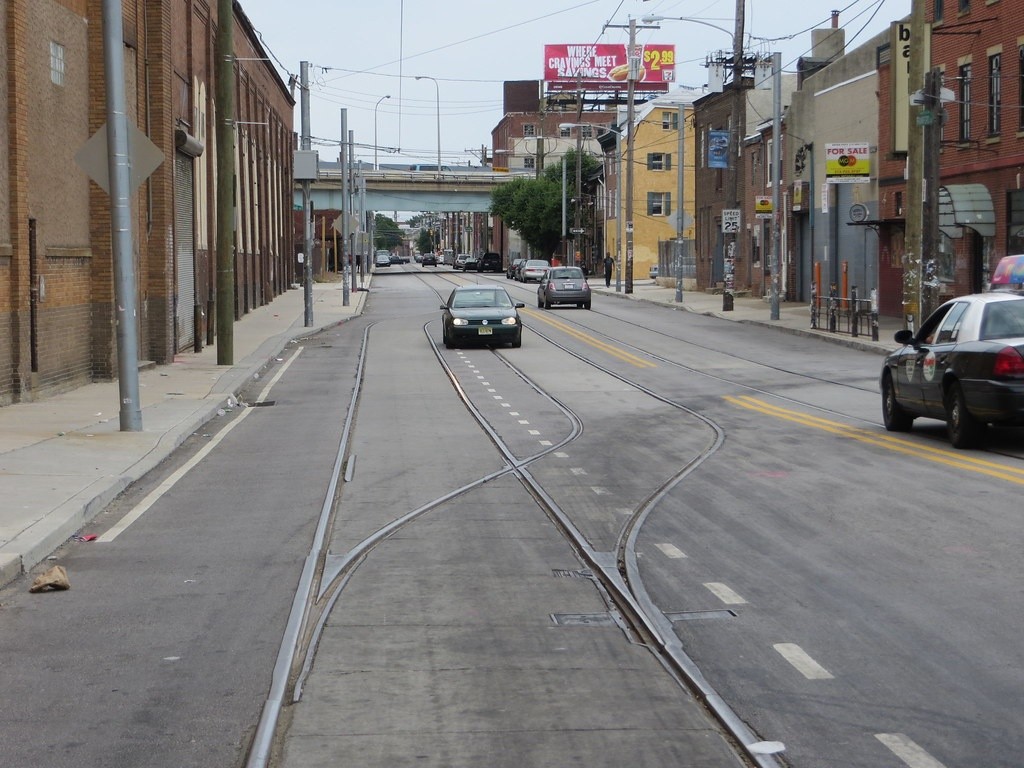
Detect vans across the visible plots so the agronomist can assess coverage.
[409,163,458,180]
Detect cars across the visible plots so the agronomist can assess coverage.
[462,257,479,272]
[877,252,1024,450]
[439,283,525,350]
[374,247,472,271]
[506,258,552,283]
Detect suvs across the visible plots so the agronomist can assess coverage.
[475,252,503,273]
[535,266,592,310]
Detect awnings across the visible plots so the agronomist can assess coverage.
[937,183,995,239]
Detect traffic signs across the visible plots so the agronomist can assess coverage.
[569,227,586,235]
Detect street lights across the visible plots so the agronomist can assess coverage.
[524,136,583,268]
[415,75,442,180]
[558,121,636,293]
[374,95,392,171]
[495,148,543,260]
[639,12,745,311]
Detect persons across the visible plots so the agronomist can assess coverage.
[603,252,615,288]
[580,258,585,270]
[356,255,360,273]
